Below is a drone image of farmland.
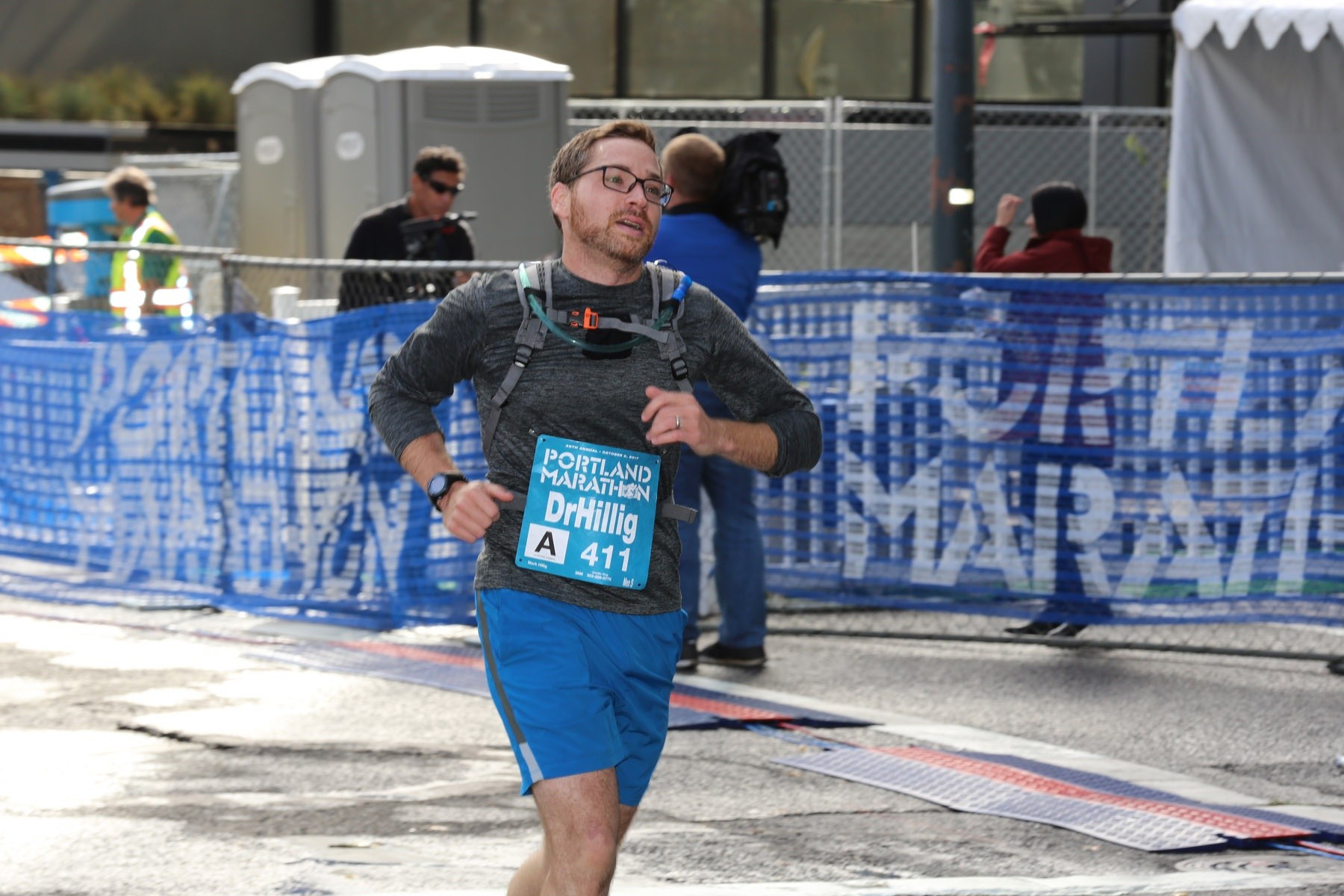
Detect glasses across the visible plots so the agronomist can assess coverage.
[561,163,675,213]
[419,173,461,197]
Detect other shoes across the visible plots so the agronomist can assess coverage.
[696,640,767,668]
[674,634,699,672]
[1001,604,1115,646]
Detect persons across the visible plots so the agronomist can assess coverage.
[324,148,476,595]
[640,134,767,671]
[98,169,197,320]
[974,181,1117,634]
[367,121,822,896]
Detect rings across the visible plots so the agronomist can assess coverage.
[676,414,680,430]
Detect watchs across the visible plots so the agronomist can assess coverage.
[427,473,469,511]
